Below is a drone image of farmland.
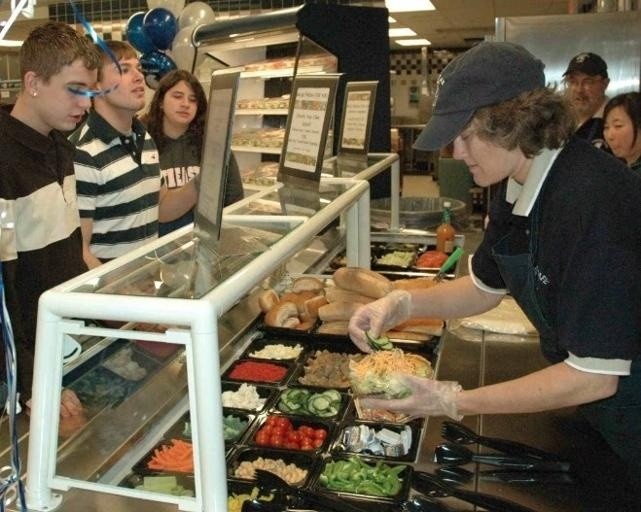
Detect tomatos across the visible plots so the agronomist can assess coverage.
[255,414,327,452]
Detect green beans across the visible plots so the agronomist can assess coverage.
[320,456,407,499]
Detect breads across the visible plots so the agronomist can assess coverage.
[259,266,444,341]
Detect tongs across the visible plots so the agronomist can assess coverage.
[400,470,537,511]
[431,420,571,474]
[241,468,364,511]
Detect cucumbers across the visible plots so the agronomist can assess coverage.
[365,329,393,352]
[277,387,342,417]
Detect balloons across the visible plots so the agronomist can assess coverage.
[125,0,215,91]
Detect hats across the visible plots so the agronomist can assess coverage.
[411,40,546,153]
[561,52,607,78]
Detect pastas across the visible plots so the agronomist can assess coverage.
[352,348,432,387]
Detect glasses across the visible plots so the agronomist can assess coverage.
[565,77,603,88]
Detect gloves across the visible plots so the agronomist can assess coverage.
[360,368,464,424]
[347,288,414,354]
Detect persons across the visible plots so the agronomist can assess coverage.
[61,39,200,273]
[601,91,641,176]
[137,69,243,237]
[0,20,90,421]
[561,51,611,149]
[347,38,640,472]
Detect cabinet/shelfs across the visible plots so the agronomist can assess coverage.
[24,154,465,512]
[188,0,392,199]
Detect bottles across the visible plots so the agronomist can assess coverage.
[436,202,455,256]
